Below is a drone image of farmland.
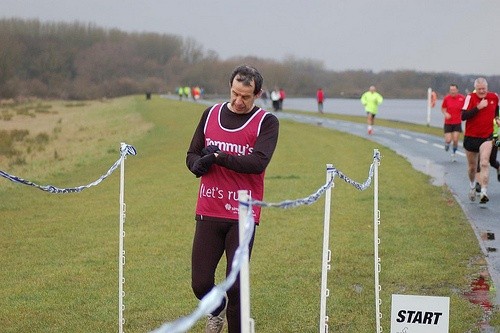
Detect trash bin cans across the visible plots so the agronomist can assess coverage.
[145,92,151,99]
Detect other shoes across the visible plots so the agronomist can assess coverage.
[451,154,456,162]
[476,182,481,192]
[497,173,500,182]
[445,144,449,151]
[468,187,476,201]
[368,129,374,134]
[479,193,489,204]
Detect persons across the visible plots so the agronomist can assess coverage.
[440,84,467,161]
[459,78,500,204]
[360,86,383,134]
[472,101,500,192]
[185,65,280,333]
[271,86,284,112]
[177,84,205,102]
[315,87,325,113]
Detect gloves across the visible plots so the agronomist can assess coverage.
[192,153,216,178]
[200,145,220,158]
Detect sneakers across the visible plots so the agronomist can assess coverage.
[206,291,228,333]
